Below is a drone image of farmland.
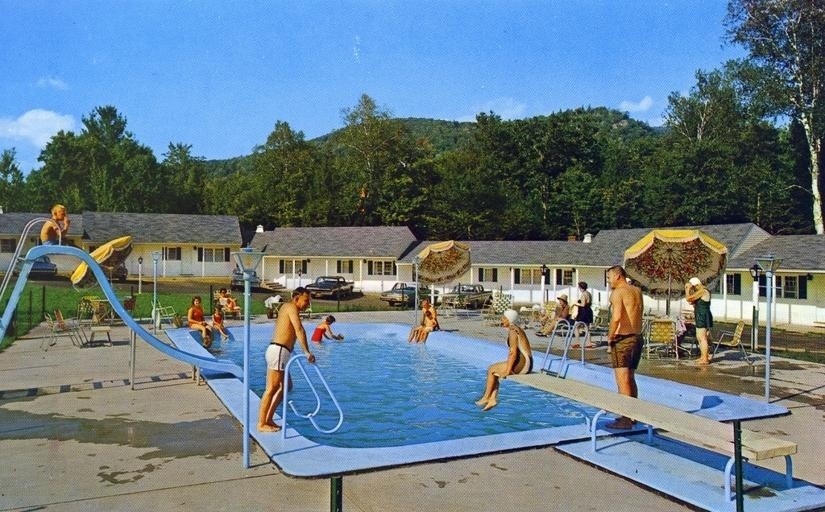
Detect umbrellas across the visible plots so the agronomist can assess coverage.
[409,239,474,286]
[68,234,134,293]
[622,228,729,317]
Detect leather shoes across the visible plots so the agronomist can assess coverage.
[535,332,547,337]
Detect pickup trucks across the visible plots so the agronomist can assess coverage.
[305,275,353,300]
[380,282,438,307]
[442,283,493,309]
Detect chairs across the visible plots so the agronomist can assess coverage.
[641,309,753,365]
[150,298,182,329]
[214,288,243,320]
[38,306,89,349]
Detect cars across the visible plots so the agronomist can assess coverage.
[110,262,128,281]
[13,254,57,281]
[231,269,261,291]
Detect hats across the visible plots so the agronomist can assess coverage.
[689,277,700,287]
[504,309,518,325]
[557,295,568,303]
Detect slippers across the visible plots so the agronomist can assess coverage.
[585,345,591,349]
[571,344,580,348]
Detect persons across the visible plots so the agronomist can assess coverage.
[256,282,316,432]
[208,303,230,340]
[534,293,570,336]
[40,204,71,245]
[187,295,213,342]
[473,307,533,411]
[416,312,437,343]
[407,300,436,344]
[216,287,241,312]
[570,281,593,348]
[684,277,712,365]
[311,314,344,345]
[604,264,646,430]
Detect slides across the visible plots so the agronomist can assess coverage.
[63,243,244,378]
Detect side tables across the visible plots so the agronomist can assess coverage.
[88,326,113,347]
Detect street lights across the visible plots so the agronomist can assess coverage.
[233,245,264,471]
[753,255,783,405]
[150,251,163,335]
[539,264,549,309]
